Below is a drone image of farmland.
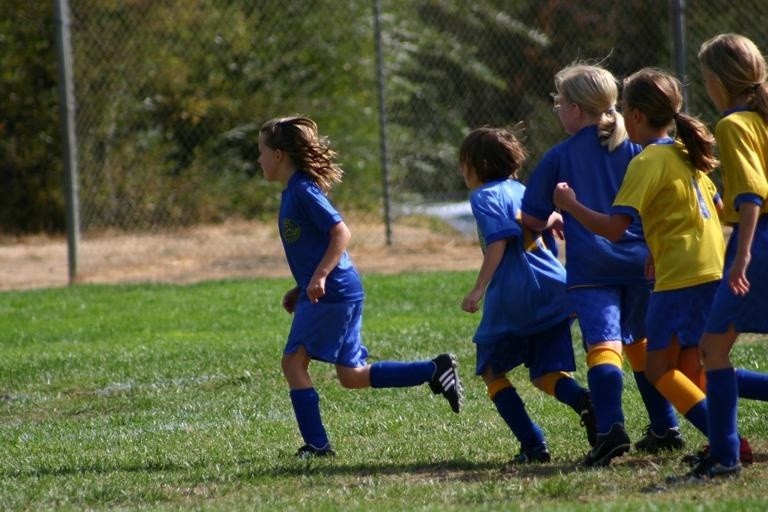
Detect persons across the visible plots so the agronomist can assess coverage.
[551,62,767,466]
[255,112,462,458]
[520,61,688,472]
[667,26,766,483]
[458,121,593,469]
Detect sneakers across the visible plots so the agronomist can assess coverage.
[428,353,464,412]
[665,453,746,489]
[634,423,685,454]
[576,387,632,467]
[681,437,752,470]
[510,442,551,465]
[294,442,336,457]
